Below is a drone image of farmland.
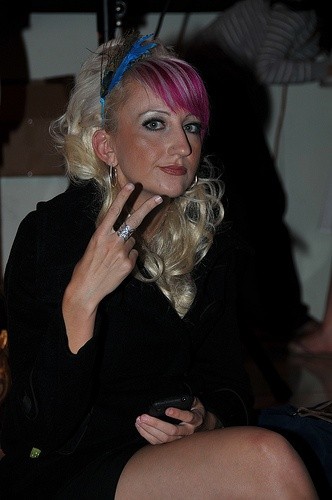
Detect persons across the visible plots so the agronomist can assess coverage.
[167,0,332,360]
[290,270,332,353]
[0,28,329,500]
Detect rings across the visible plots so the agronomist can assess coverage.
[115,223,134,242]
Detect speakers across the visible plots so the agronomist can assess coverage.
[97,0,137,46]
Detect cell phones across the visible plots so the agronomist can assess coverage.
[147,396,193,425]
